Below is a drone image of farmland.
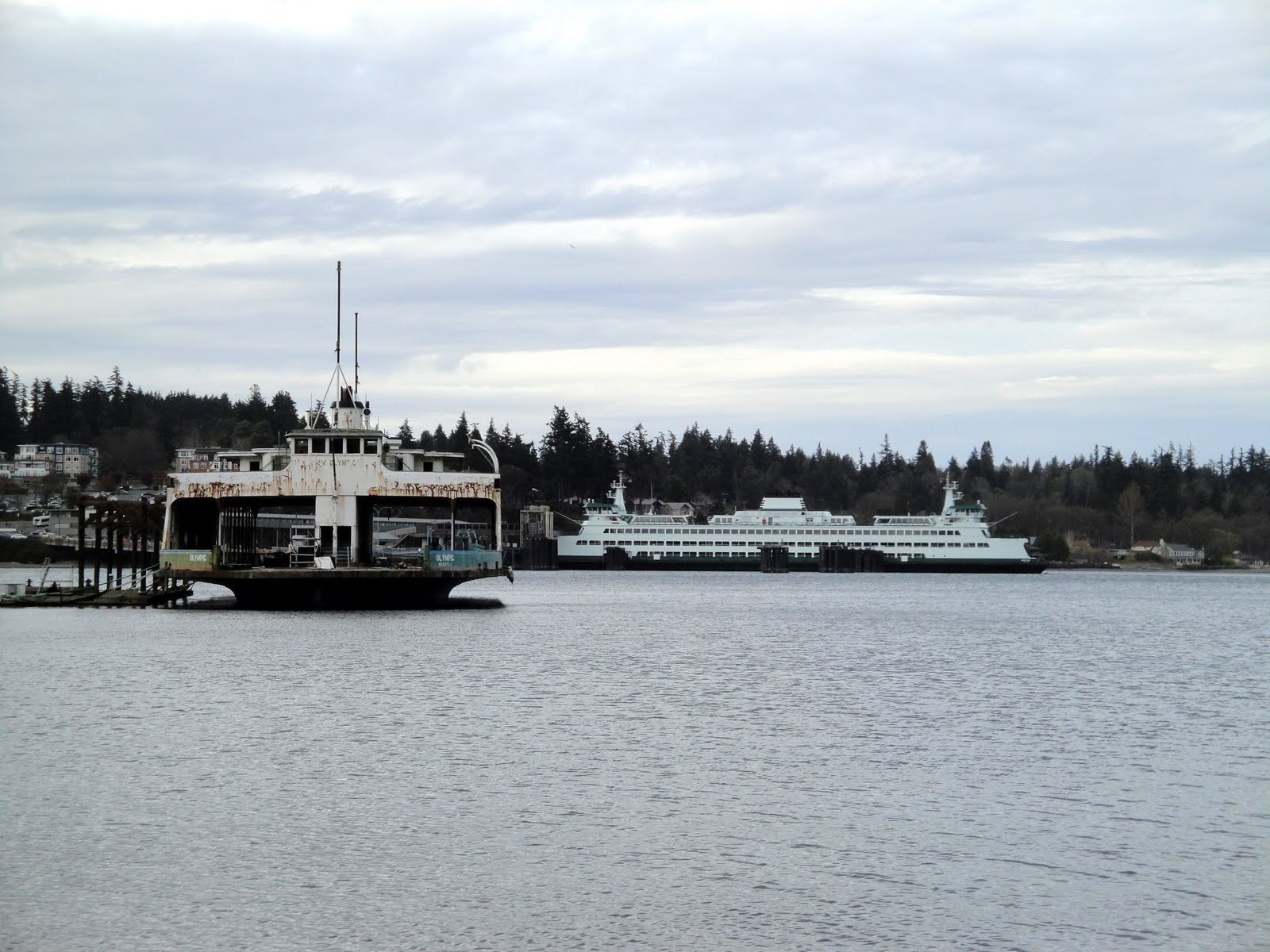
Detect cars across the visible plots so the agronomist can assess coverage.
[0,485,163,540]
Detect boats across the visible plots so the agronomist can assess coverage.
[156,258,516,611]
[557,469,1050,573]
